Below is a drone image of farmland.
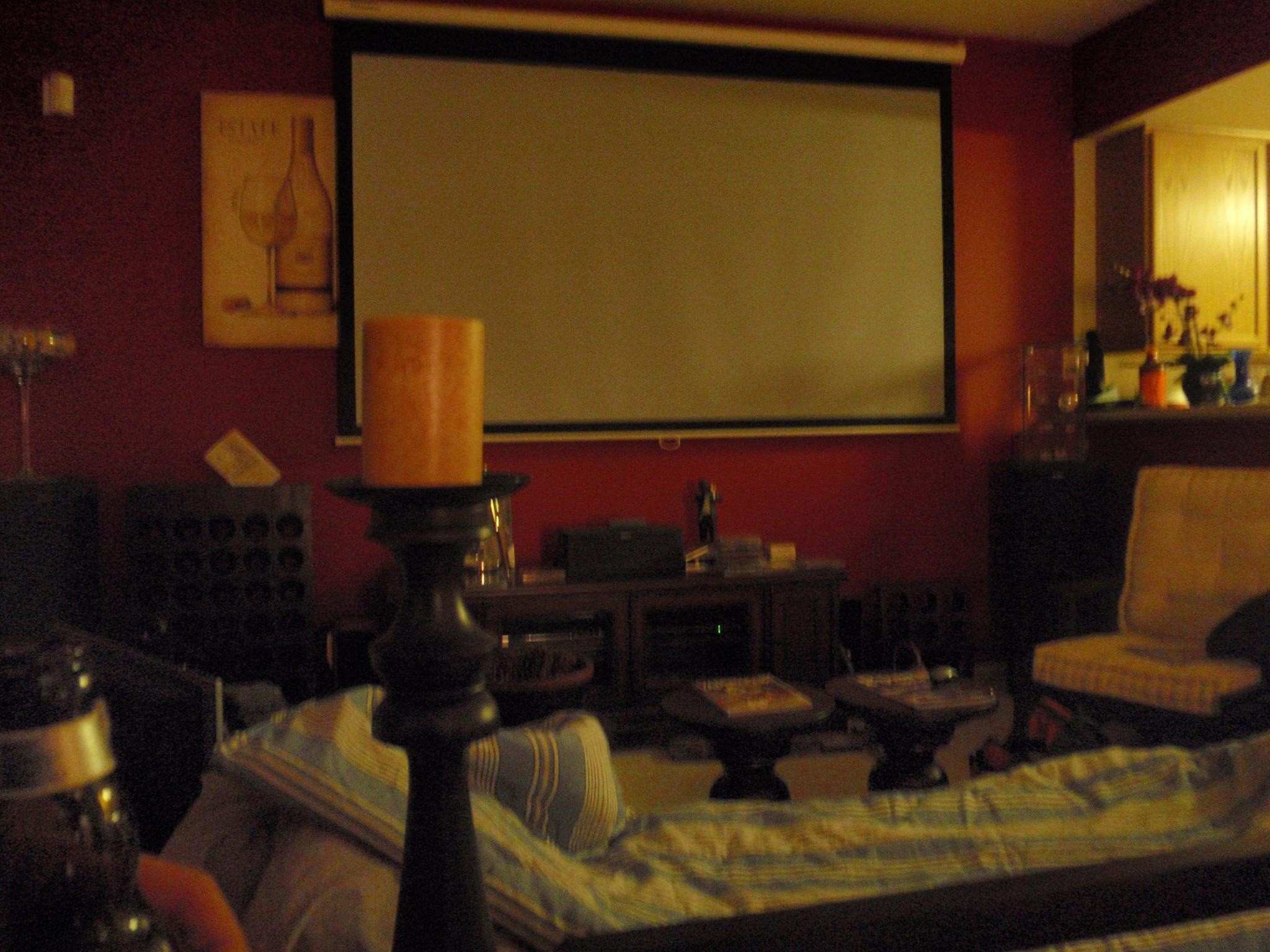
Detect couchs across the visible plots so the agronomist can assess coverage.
[215,688,1270,952]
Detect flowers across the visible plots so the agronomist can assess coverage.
[1176,289,1244,357]
[1105,265,1194,357]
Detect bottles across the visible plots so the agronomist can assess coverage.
[1229,350,1262,406]
[1139,348,1163,406]
[1,620,174,952]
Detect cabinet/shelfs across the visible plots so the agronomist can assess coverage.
[1096,126,1270,363]
[464,566,846,750]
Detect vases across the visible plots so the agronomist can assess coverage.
[1140,307,1163,407]
[1177,354,1231,409]
[1227,349,1259,407]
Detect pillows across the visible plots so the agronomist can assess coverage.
[163,772,402,952]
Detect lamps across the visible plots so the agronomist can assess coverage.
[0,327,77,481]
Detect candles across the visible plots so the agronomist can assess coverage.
[364,317,485,486]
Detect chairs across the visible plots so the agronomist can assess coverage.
[1007,464,1270,760]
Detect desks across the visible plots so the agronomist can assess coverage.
[837,675,998,790]
[673,680,836,801]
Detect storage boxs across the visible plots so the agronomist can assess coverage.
[203,428,281,487]
